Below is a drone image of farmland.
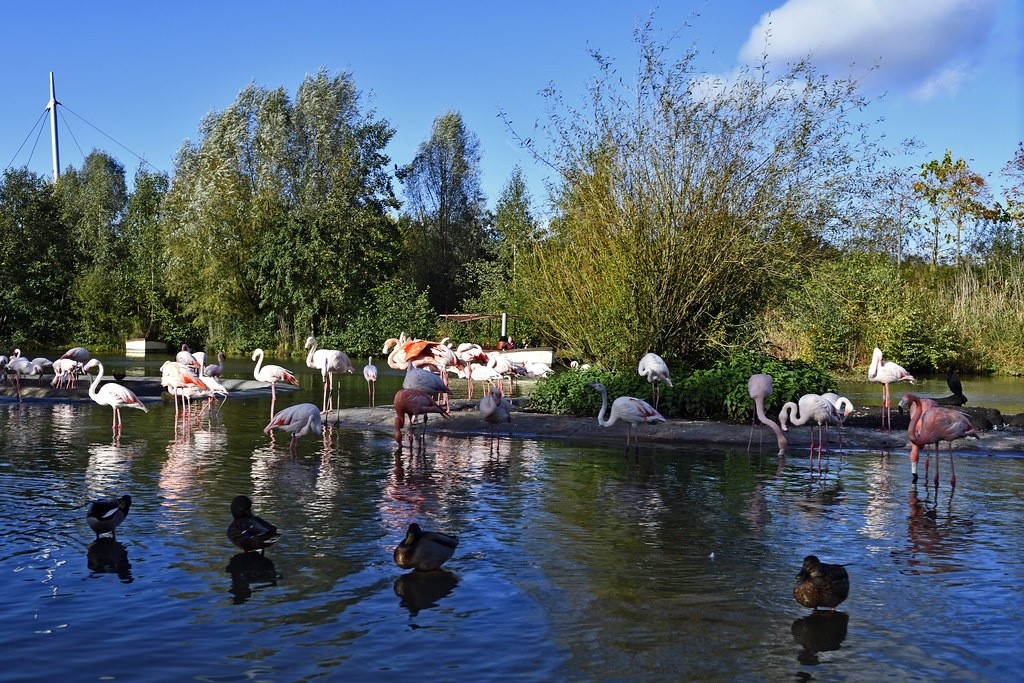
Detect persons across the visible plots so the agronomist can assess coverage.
[502,336,530,350]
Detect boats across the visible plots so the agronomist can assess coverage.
[124,336,169,354]
[438,311,558,370]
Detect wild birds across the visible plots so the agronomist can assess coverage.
[404,361,455,444]
[83,358,150,428]
[819,393,854,461]
[897,393,981,487]
[779,393,842,467]
[793,556,850,612]
[0,347,88,400]
[226,495,283,554]
[86,493,133,538]
[479,388,517,450]
[393,387,456,449]
[639,353,674,411]
[263,404,326,460]
[867,348,916,432]
[746,373,789,459]
[589,382,666,445]
[159,330,591,416]
[393,523,459,571]
